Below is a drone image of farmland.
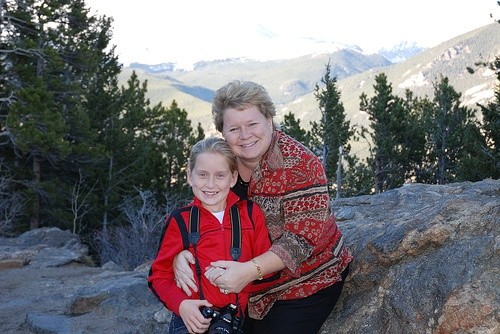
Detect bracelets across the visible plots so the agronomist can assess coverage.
[251,259,263,280]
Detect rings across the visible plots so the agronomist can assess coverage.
[224,290,227,294]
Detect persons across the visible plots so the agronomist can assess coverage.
[172,80,353,334]
[148,138,279,334]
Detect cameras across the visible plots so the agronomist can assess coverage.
[200,302,240,334]
